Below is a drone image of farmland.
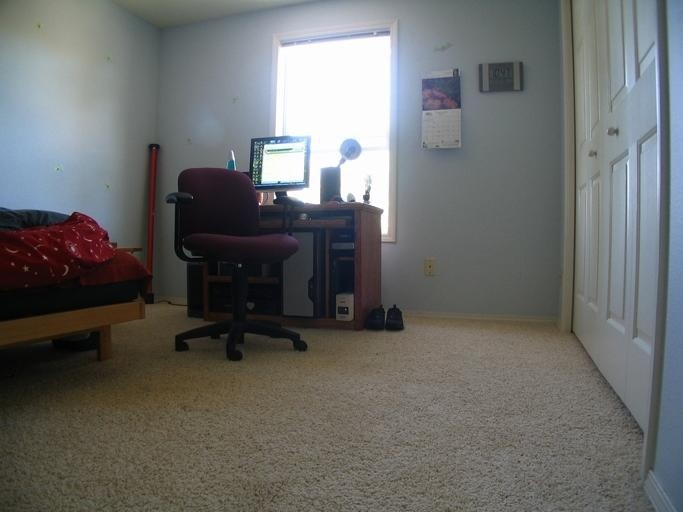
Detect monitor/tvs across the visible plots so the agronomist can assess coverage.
[249,134,311,204]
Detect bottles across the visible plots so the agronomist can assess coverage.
[225,150,236,171]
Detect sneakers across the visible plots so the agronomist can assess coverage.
[366,305,385,330]
[386,304,403,328]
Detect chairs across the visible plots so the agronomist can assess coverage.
[166,167,307,360]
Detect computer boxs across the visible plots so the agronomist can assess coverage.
[329,229,354,320]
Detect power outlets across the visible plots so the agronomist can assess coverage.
[424,258,440,276]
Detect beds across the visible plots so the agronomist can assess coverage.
[0,207,153,361]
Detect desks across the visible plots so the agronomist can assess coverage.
[203,202,383,331]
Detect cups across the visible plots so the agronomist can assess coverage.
[256,192,269,205]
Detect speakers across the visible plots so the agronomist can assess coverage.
[321,167,341,203]
